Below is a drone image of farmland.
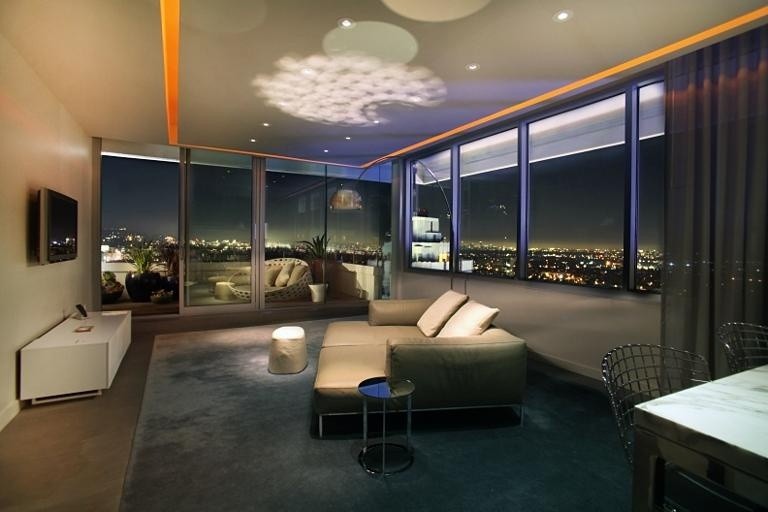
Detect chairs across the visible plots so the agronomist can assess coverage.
[228,257,313,302]
[599,318,766,470]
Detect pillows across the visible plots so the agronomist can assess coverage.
[264,261,308,288]
[417,289,499,339]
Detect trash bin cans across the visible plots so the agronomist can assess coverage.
[308,283,329,303]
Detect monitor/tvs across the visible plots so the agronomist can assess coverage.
[37,187,79,267]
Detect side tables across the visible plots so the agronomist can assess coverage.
[360,376,415,479]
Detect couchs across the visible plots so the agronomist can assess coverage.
[313,298,528,441]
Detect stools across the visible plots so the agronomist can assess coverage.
[270,326,309,373]
[208,275,236,301]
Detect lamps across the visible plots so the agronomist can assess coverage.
[327,151,455,289]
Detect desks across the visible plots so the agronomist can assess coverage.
[633,366,768,511]
[17,307,132,405]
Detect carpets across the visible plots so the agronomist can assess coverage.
[122,315,662,512]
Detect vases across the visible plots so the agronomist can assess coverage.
[309,282,327,302]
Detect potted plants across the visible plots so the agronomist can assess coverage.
[295,233,339,286]
[100,236,163,303]
